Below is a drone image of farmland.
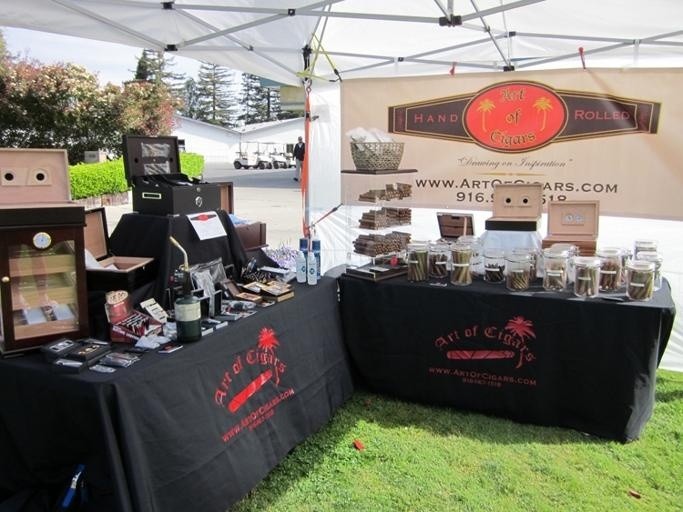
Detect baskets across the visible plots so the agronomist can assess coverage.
[350,142,404,170]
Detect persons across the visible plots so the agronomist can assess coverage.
[293,137,305,181]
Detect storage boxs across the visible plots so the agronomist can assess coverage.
[122,134,269,254]
[485,182,601,257]
[83,207,155,293]
[0,145,84,225]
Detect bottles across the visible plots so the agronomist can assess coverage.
[294,238,322,285]
[406,234,662,302]
[174,264,200,341]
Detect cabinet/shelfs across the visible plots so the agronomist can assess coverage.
[2,228,91,350]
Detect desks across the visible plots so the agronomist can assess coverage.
[2,263,675,509]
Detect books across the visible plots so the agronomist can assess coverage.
[262,292,295,302]
